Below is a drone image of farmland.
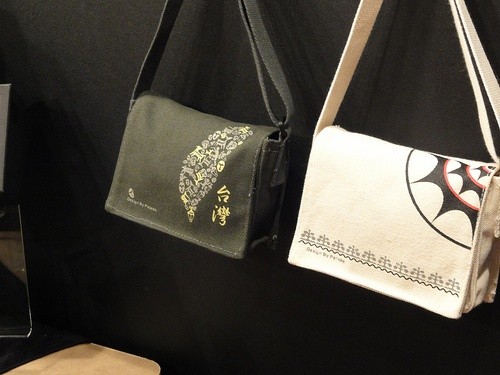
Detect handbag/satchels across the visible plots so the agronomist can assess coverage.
[287,124,499,319]
[104,88,289,260]
